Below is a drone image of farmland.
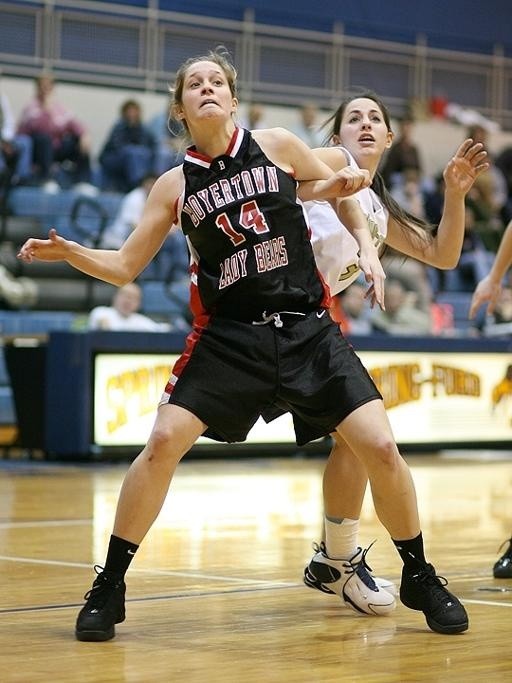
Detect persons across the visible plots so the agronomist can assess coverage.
[292,100,333,150]
[240,104,267,130]
[329,118,512,337]
[1,74,189,333]
[292,91,491,616]
[467,220,512,580]
[15,42,469,641]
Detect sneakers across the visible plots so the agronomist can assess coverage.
[75,563,126,641]
[399,563,468,634]
[303,546,398,616]
[493,538,511,578]
[304,541,398,596]
